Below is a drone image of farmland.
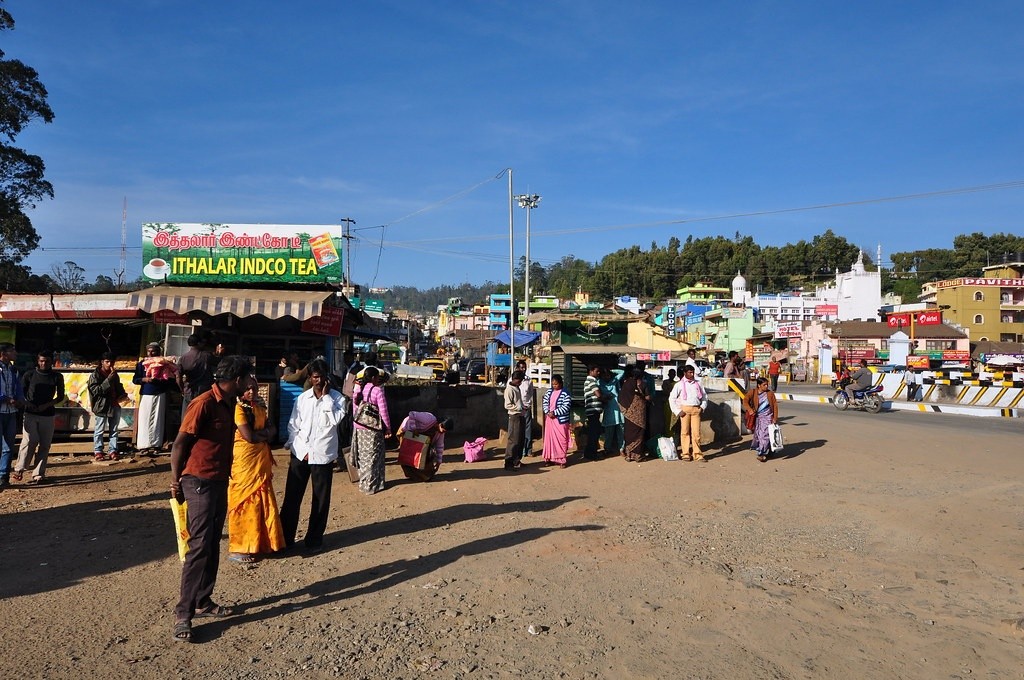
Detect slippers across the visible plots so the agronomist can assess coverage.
[33,475,45,481]
[226,552,255,562]
[13,471,23,480]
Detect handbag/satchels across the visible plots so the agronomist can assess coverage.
[768,423,784,452]
[398,431,430,470]
[658,437,679,460]
[653,434,665,458]
[353,400,382,430]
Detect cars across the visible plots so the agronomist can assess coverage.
[461,358,490,384]
[418,357,448,381]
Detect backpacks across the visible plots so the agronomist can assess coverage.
[463,437,487,463]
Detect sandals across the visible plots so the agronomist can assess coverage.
[153,449,158,455]
[696,457,708,463]
[682,452,692,461]
[625,455,631,462]
[634,455,641,461]
[140,448,149,455]
[173,617,193,642]
[110,452,118,460]
[95,453,105,461]
[194,602,233,616]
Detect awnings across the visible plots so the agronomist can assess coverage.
[127,287,335,322]
[493,330,541,348]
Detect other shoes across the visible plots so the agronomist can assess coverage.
[514,462,527,468]
[583,449,624,460]
[273,544,296,555]
[302,541,323,553]
[505,466,518,471]
[544,462,551,467]
[757,454,769,461]
[560,464,566,468]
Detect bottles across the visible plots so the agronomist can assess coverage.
[52,351,71,367]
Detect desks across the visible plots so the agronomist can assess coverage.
[54,407,83,432]
[121,407,135,409]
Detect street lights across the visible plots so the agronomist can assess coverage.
[512,193,543,356]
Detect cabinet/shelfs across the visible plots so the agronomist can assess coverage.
[163,323,324,378]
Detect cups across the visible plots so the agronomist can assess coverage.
[149,258,171,273]
[319,249,330,258]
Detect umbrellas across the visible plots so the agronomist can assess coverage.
[986,354,1024,369]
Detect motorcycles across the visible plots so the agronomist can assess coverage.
[832,374,885,414]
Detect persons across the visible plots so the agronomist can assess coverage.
[178,332,214,423]
[685,350,783,462]
[903,365,918,402]
[845,359,872,405]
[133,341,180,455]
[503,361,535,472]
[228,378,287,564]
[342,350,391,495]
[584,360,709,463]
[279,359,346,557]
[452,359,462,384]
[275,350,308,381]
[170,354,249,642]
[0,341,65,491]
[542,374,571,469]
[90,351,128,462]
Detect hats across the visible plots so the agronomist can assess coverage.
[146,342,159,347]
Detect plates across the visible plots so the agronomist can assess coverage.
[143,263,170,279]
[322,256,334,262]
